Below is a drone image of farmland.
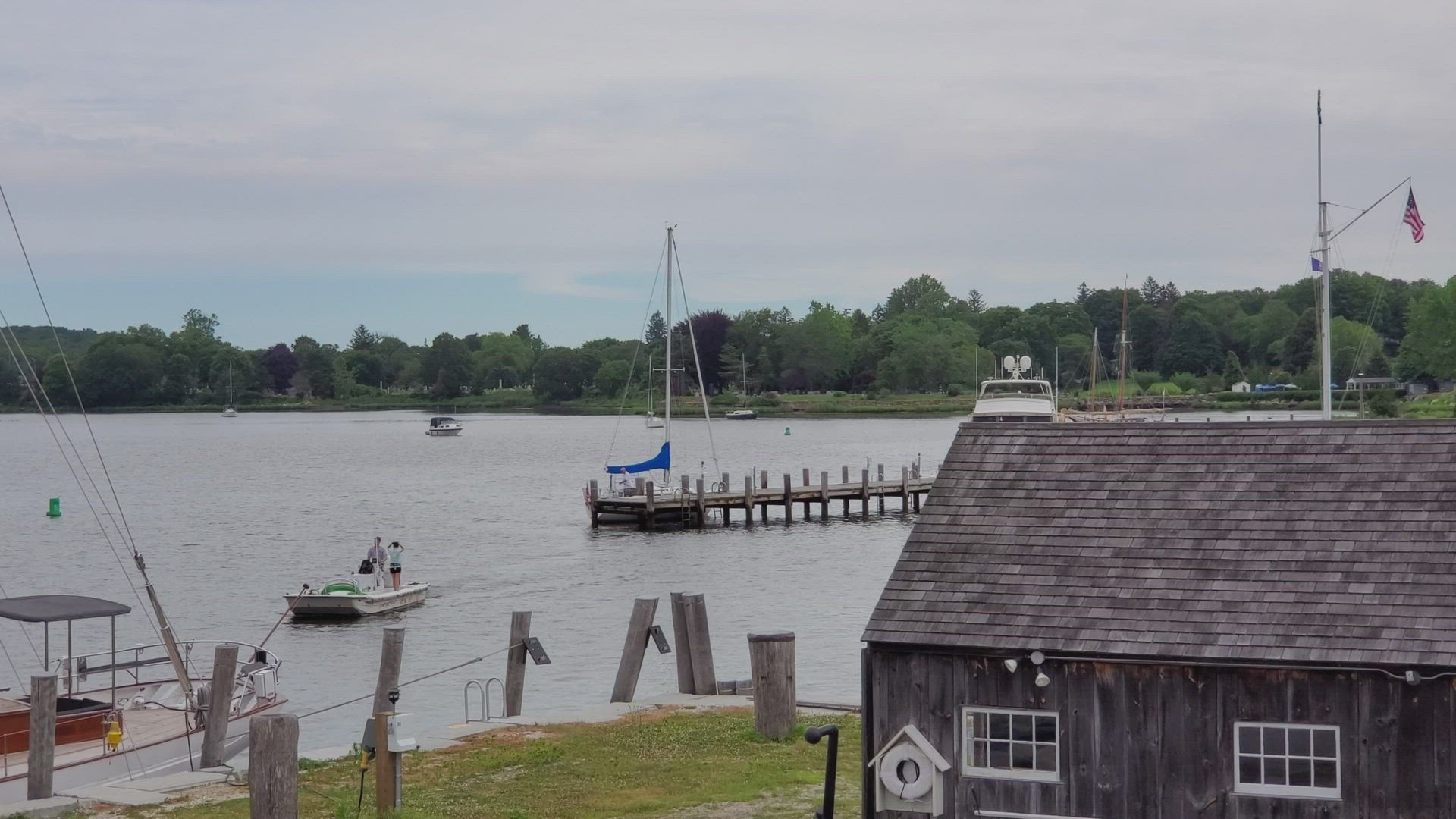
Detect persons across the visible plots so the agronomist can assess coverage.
[367,537,387,586]
[387,542,404,590]
[619,467,632,489]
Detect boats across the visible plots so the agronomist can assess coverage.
[967,344,1061,423]
[282,534,431,617]
[424,415,464,436]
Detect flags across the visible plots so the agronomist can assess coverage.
[1402,186,1425,243]
[1312,257,1331,273]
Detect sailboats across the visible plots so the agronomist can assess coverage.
[1058,273,1174,422]
[0,180,290,807]
[725,351,760,420]
[220,361,238,418]
[642,355,666,429]
[580,218,722,525]
